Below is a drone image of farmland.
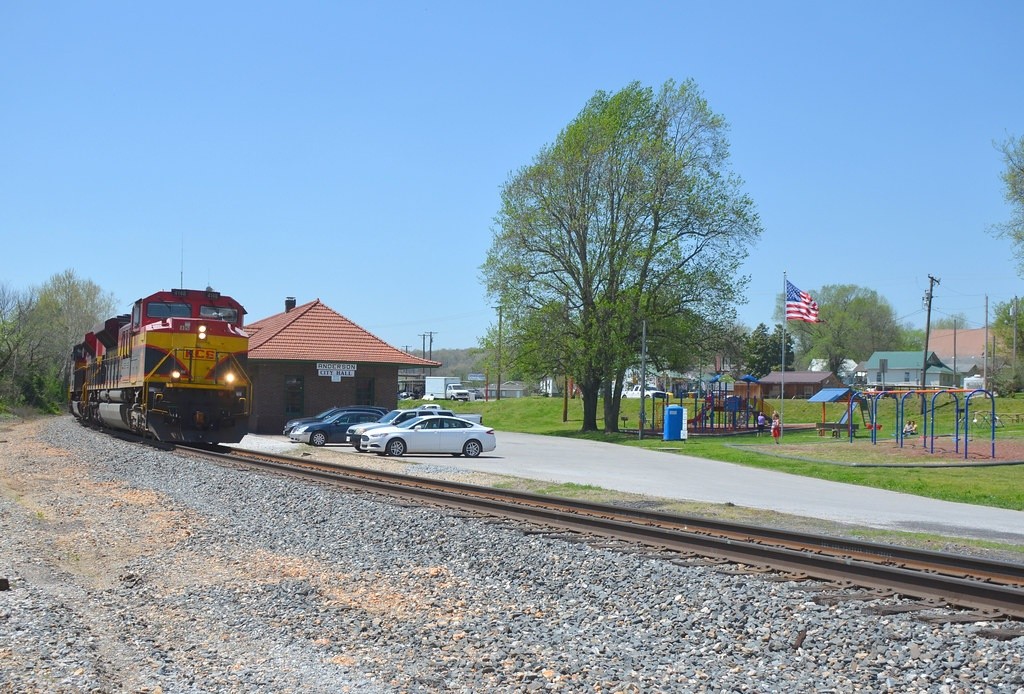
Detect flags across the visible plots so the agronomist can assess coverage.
[786,280,825,322]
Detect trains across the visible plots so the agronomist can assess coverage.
[69,287,254,447]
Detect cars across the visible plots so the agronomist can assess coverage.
[289,411,383,447]
[332,405,390,415]
[359,415,497,458]
[467,390,484,399]
[282,406,385,440]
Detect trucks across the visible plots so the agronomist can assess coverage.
[425,376,469,402]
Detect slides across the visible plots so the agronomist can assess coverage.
[688,404,710,424]
[839,399,858,426]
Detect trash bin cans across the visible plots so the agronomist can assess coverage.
[664,404,688,441]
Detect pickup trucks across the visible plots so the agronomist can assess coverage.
[345,409,483,454]
[621,385,666,398]
[416,404,440,411]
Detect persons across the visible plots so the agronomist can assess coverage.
[903,420,913,433]
[911,421,918,435]
[772,413,780,444]
[757,412,765,437]
